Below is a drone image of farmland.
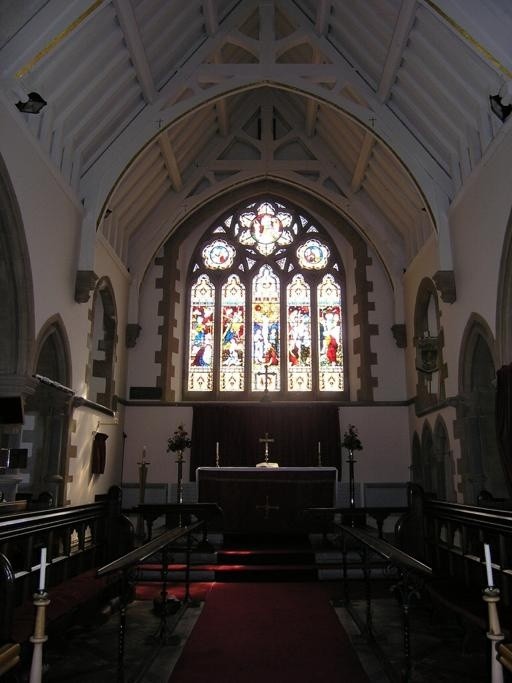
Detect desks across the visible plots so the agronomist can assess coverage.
[194,464,343,550]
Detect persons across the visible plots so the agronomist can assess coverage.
[193,328,340,366]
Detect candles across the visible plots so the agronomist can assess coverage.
[318,441,321,456]
[482,543,495,588]
[216,442,219,458]
[38,547,47,591]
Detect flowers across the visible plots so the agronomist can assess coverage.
[164,422,191,453]
[341,423,364,452]
